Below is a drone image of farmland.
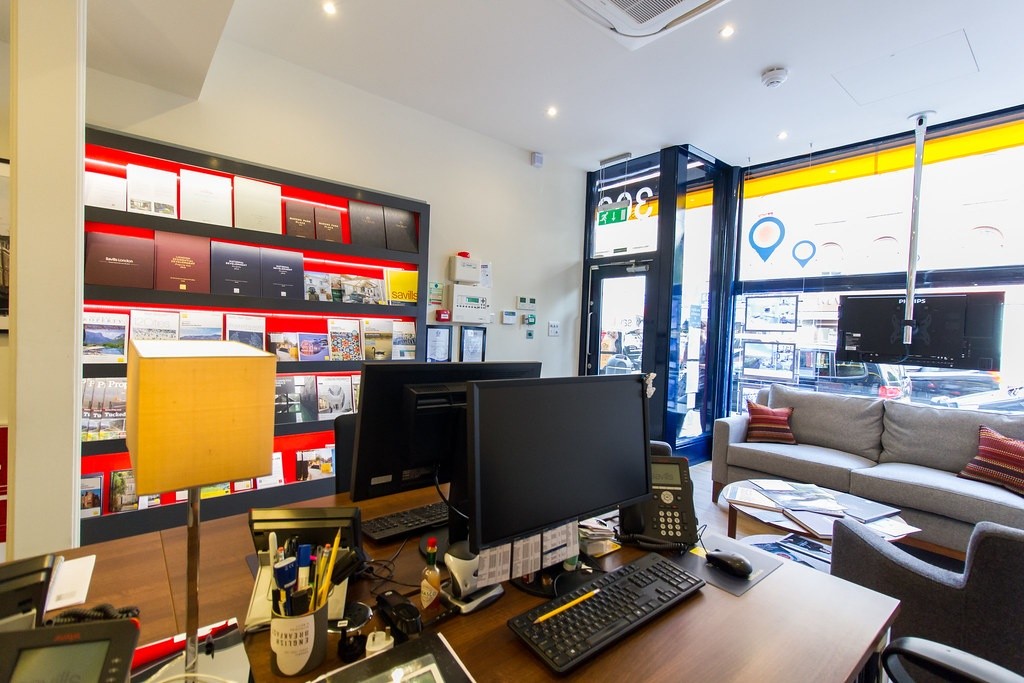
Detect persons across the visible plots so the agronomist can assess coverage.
[786,535,832,554]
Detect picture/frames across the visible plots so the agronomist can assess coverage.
[426,325,452,362]
[460,326,487,362]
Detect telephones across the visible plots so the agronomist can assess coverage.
[619,456,699,552]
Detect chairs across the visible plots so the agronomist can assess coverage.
[826,519,1024,683]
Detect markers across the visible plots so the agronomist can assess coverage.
[300,544,310,591]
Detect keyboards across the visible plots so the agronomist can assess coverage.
[506,553,706,675]
[361,501,450,544]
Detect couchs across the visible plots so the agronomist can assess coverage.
[712,383,1024,563]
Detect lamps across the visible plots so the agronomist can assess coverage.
[124,340,277,682]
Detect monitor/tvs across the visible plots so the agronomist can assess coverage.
[838,290,1005,371]
[351,361,542,502]
[465,373,652,597]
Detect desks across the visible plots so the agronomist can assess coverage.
[44,484,903,683]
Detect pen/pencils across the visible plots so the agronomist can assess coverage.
[536,586,601,624]
[268,527,344,616]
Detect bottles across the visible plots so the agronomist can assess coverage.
[420,538,440,612]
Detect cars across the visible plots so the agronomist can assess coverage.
[908,366,1024,413]
[733,334,781,350]
[731,342,912,412]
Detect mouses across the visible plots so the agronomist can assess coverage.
[705,549,752,577]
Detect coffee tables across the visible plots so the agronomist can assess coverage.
[723,479,902,578]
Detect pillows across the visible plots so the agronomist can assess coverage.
[957,425,1024,497]
[745,400,798,445]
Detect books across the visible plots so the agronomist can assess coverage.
[782,508,859,539]
[295,444,335,481]
[776,532,832,563]
[275,375,318,423]
[82,377,126,442]
[360,318,402,360]
[297,332,330,361]
[833,493,902,524]
[328,319,362,361]
[226,314,265,350]
[180,310,224,341]
[81,311,129,363]
[351,374,361,413]
[726,480,848,518]
[109,470,138,512]
[81,472,104,517]
[270,332,299,361]
[139,451,285,509]
[131,310,179,340]
[392,321,416,360]
[317,376,353,421]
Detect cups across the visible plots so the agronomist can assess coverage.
[270,600,329,676]
